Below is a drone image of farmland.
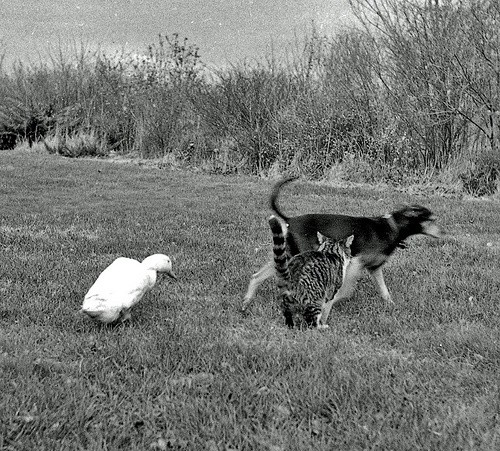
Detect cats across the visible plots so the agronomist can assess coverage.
[267,214,357,331]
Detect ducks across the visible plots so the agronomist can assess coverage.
[78,253,180,329]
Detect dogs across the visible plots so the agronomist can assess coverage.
[238,175,449,307]
